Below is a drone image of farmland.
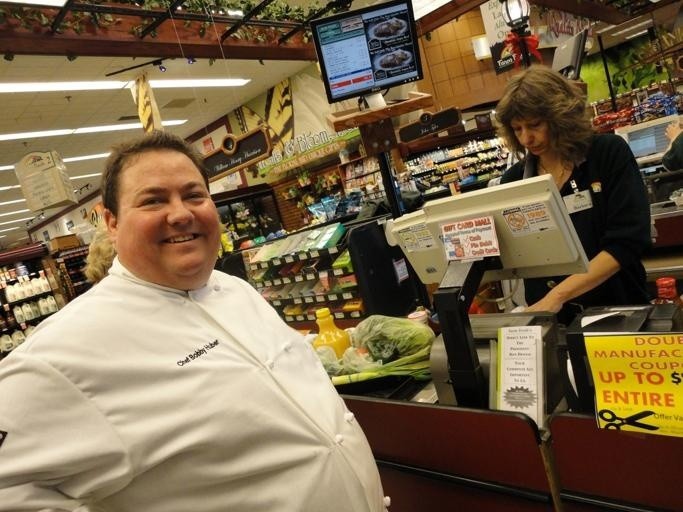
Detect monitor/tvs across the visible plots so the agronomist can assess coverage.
[615,114,680,165]
[423,173,589,285]
[310,0,423,104]
[552,28,588,81]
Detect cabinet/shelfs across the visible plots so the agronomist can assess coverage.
[0,289,62,338]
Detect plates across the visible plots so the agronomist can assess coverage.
[368,17,407,41]
[374,49,411,72]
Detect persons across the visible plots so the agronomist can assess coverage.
[83,227,117,285]
[492,64,653,314]
[662,122,682,171]
[0,134,389,512]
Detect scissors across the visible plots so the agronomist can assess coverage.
[599,409,659,430]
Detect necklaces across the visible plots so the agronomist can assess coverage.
[539,163,567,188]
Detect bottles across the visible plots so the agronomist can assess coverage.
[311,308,352,360]
[653,277,682,306]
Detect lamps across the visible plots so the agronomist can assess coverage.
[499,0,532,32]
